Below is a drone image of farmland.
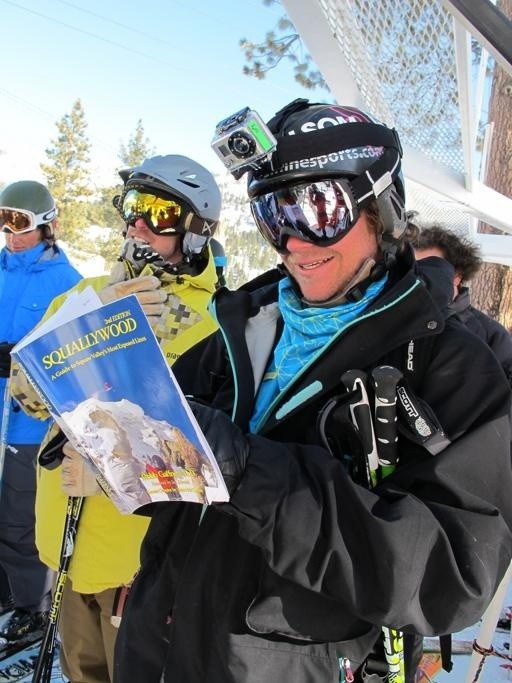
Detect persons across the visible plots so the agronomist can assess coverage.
[7,151,227,681]
[129,203,182,229]
[0,208,29,230]
[0,178,84,649]
[409,225,511,384]
[106,96,512,681]
[255,181,350,238]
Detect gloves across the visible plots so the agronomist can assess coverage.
[99,263,167,327]
[0,341,12,380]
[61,441,102,497]
[185,400,248,499]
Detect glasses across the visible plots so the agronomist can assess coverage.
[0,207,38,235]
[121,188,190,235]
[250,177,361,254]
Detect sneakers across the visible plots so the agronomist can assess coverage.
[0,609,48,643]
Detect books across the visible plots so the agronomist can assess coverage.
[9,283,236,525]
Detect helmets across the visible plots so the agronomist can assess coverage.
[0,180,56,228]
[209,238,226,288]
[246,98,410,264]
[118,155,220,255]
